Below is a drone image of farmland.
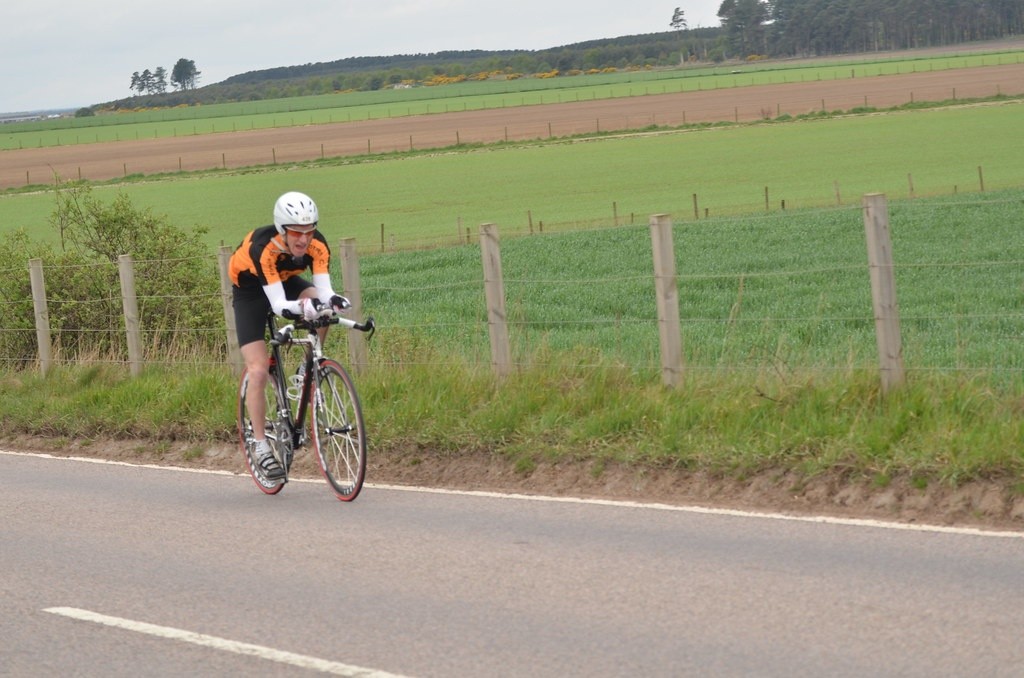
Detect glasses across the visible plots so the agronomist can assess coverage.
[286,227,317,239]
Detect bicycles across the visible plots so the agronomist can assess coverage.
[237,309,376,501]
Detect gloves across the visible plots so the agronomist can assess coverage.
[330,295,352,315]
[302,298,323,320]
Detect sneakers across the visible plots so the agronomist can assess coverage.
[255,454,286,480]
[297,367,325,404]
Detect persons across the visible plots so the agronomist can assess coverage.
[227,191,352,479]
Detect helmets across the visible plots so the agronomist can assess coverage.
[273,191,319,237]
